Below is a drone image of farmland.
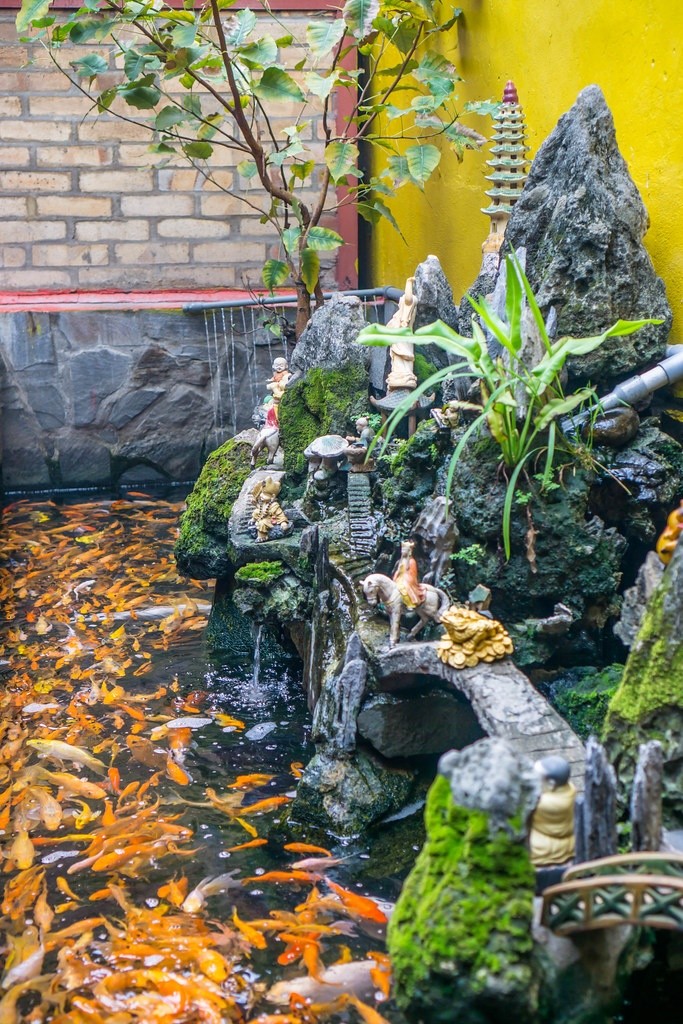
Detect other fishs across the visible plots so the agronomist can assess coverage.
[0,490,390,1022]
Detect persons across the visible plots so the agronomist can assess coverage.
[388,293,418,387]
[525,756,577,896]
[249,391,284,466]
[656,499,683,565]
[251,477,288,543]
[394,540,426,611]
[266,356,293,382]
[346,416,375,450]
[435,401,460,429]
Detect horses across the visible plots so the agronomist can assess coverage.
[249,425,280,471]
[359,573,449,651]
